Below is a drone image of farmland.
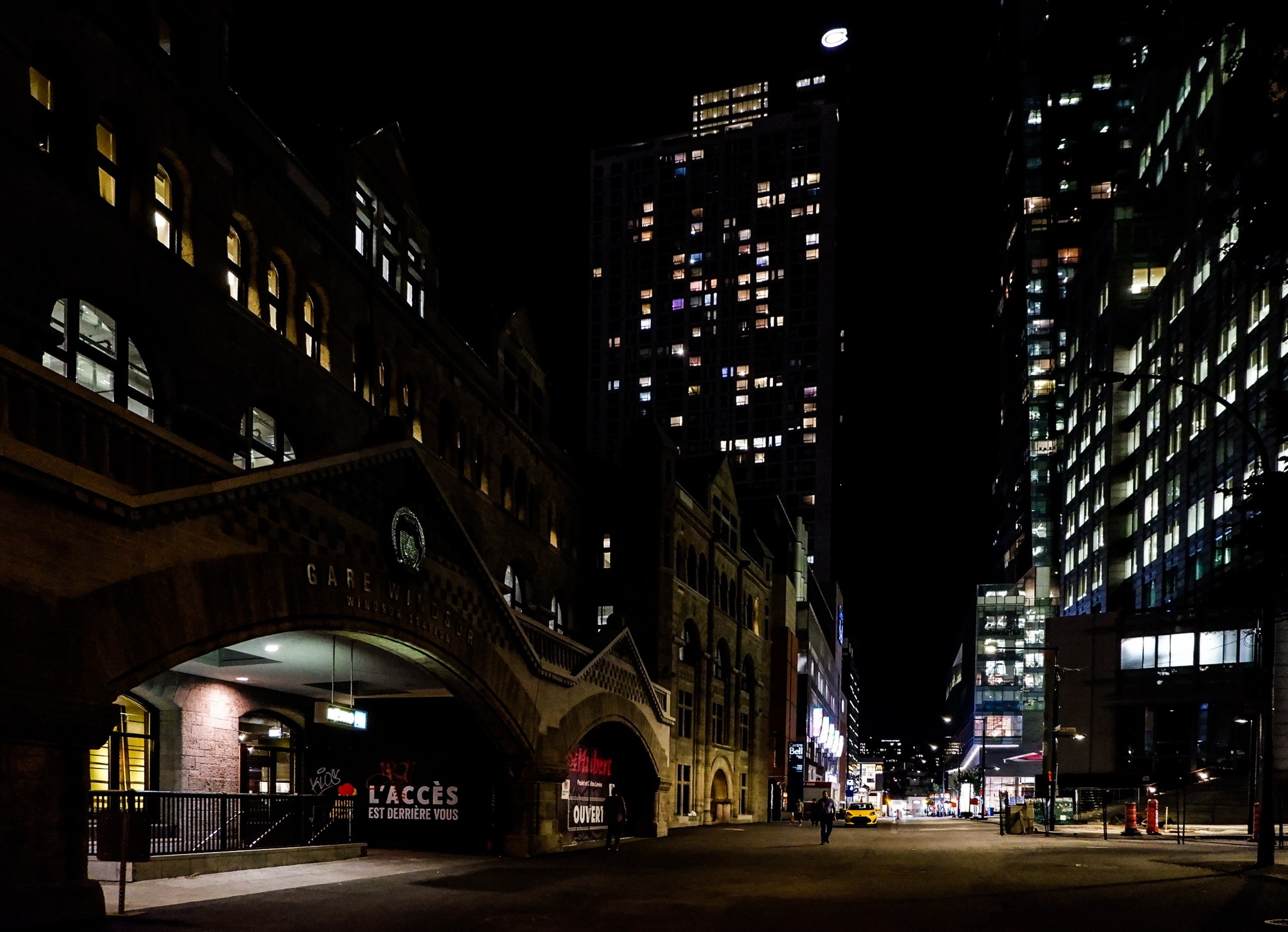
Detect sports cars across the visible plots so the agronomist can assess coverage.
[842,803,881,827]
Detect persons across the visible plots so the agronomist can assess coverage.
[795,798,804,827]
[816,790,836,845]
[807,798,819,827]
[602,787,626,852]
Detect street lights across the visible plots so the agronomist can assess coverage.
[930,744,961,817]
[984,644,1087,832]
[943,715,986,819]
[1232,716,1256,833]
[1091,369,1276,868]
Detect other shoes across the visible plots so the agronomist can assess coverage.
[825,838,829,843]
[798,824,802,826]
[812,823,815,826]
[821,839,824,845]
[816,824,819,826]
